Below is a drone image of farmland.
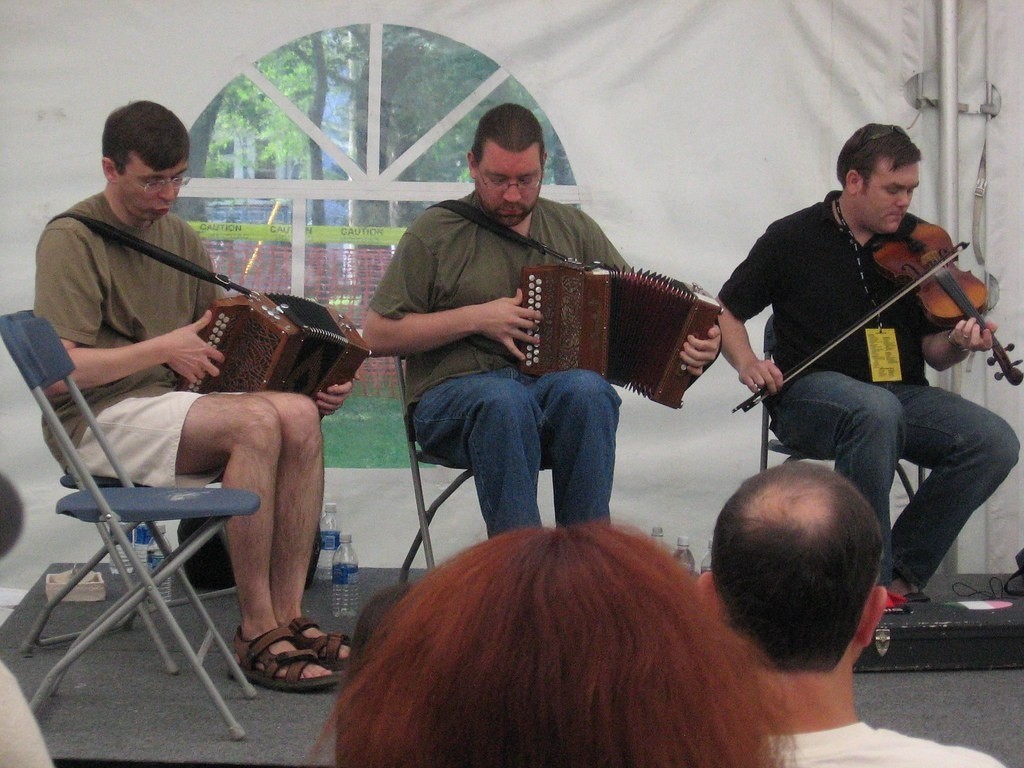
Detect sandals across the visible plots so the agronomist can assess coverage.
[226,617,354,690]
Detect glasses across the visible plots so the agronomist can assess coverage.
[477,165,542,190]
[122,164,190,194]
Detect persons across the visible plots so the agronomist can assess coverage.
[362,103,722,541]
[0,473,54,767]
[716,123,1020,609]
[310,524,797,768]
[698,463,1007,768]
[34,100,361,694]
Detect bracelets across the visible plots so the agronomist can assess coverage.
[947,329,969,353]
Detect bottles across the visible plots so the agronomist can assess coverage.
[651,527,662,543]
[330,533,359,617]
[134,520,153,574]
[109,521,134,574]
[147,524,170,603]
[701,540,713,574]
[316,503,341,586]
[672,537,695,575]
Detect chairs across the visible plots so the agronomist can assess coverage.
[0,308,261,741]
[394,354,553,577]
[761,314,930,602]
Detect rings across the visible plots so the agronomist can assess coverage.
[963,334,969,338]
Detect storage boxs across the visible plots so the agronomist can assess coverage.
[46,572,107,604]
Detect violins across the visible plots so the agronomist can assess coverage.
[869,210,1024,387]
